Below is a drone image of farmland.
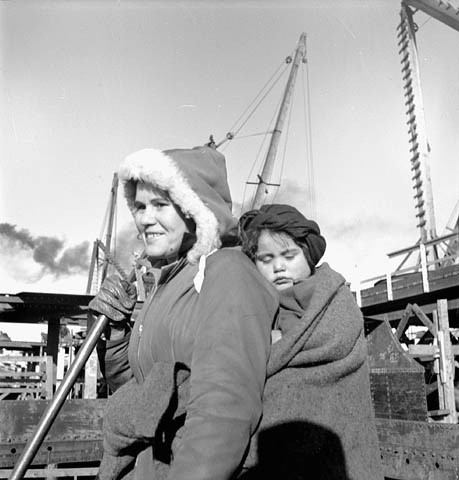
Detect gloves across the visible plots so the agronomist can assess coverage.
[87,274,137,333]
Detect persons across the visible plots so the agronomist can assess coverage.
[238,204,385,480]
[88,146,281,480]
